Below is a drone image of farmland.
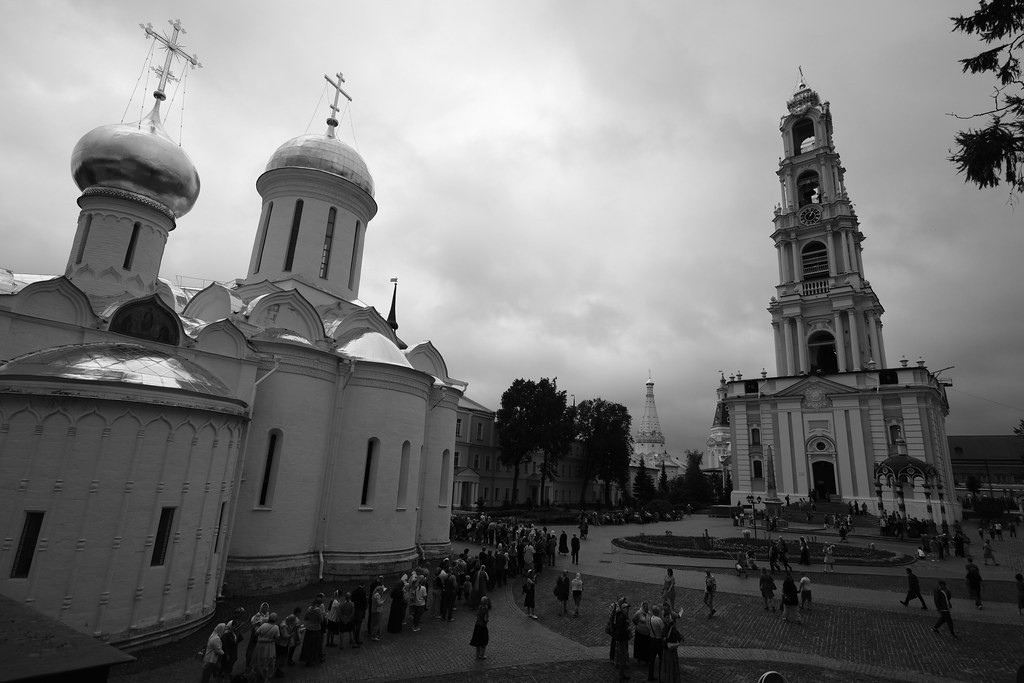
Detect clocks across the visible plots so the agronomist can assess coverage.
[798,206,822,227]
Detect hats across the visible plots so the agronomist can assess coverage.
[621,602,632,609]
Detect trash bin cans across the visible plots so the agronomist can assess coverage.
[759,670,790,683]
[742,530,751,538]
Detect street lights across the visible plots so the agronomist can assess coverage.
[746,494,762,539]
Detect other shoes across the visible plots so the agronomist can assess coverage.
[564,554,566,556]
[452,608,457,611]
[709,610,716,615]
[763,606,768,610]
[580,536,582,538]
[564,609,568,613]
[807,607,812,611]
[800,606,804,609]
[798,622,805,625]
[476,656,487,660]
[572,561,574,563]
[352,645,360,649]
[557,550,560,554]
[448,619,455,622]
[585,537,586,540]
[527,614,538,619]
[410,626,421,632]
[371,636,380,641]
[434,615,442,619]
[576,563,578,565]
[782,617,787,621]
[772,608,776,612]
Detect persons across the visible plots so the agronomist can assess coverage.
[203,491,1024,683]
